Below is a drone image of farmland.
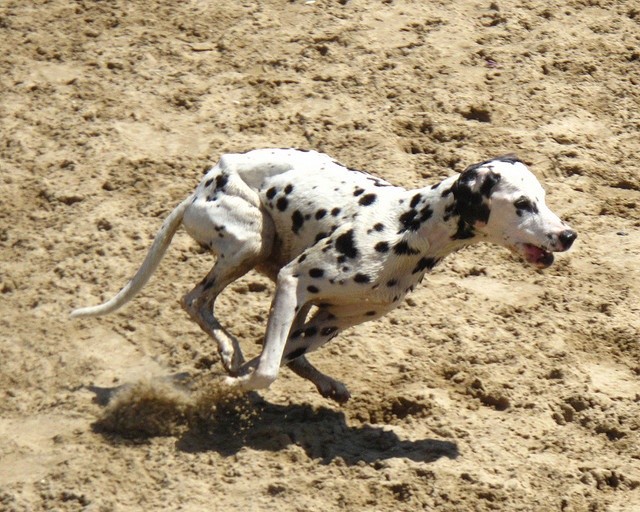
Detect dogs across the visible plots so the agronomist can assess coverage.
[68,147,577,404]
